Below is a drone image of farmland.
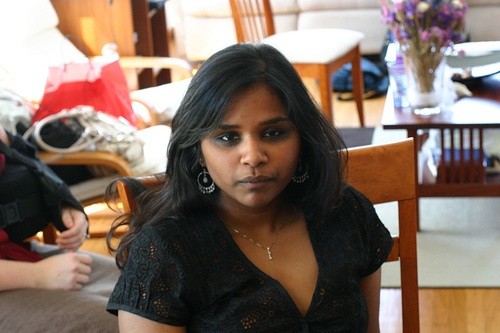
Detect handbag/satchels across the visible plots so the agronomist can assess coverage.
[331,58,390,101]
[22,104,145,183]
[29,52,139,132]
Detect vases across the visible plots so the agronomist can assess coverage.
[403,53,444,116]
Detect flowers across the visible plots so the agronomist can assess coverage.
[379,0,468,93]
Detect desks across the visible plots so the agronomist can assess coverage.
[382,67,500,197]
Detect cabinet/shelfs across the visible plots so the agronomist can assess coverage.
[50,0,171,90]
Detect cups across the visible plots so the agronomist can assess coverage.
[386,62,409,108]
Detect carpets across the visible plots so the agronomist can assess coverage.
[371,116,500,288]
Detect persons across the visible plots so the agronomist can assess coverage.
[0,120,122,306]
[104,43,395,333]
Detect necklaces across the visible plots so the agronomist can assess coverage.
[227,213,286,260]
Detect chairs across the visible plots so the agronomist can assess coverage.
[116,137,420,333]
[229,0,365,129]
[0,0,192,257]
